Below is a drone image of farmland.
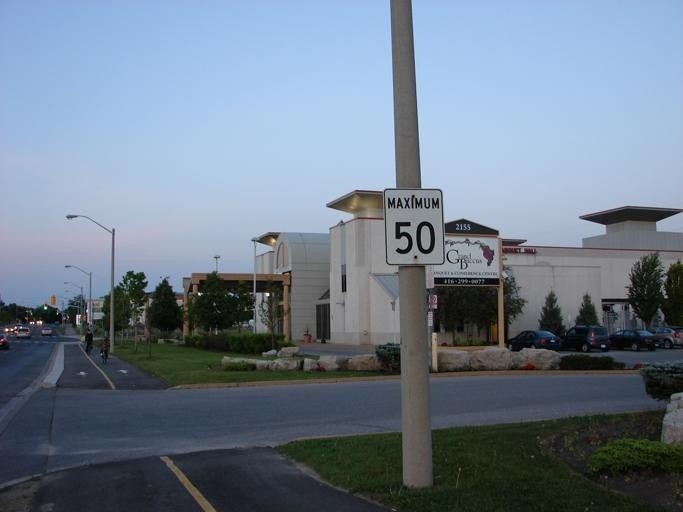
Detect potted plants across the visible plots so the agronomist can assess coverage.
[302,327,312,344]
[318,335,327,344]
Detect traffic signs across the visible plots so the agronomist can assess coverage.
[383,187,446,264]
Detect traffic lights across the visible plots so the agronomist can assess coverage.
[43,303,47,310]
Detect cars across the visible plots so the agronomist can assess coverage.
[505,324,682,352]
[0,317,54,350]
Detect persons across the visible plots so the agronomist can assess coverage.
[84,329,93,354]
[100,339,109,363]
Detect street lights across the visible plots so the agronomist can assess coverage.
[213,253,221,273]
[64,264,92,331]
[250,236,260,304]
[64,280,85,320]
[66,213,118,359]
